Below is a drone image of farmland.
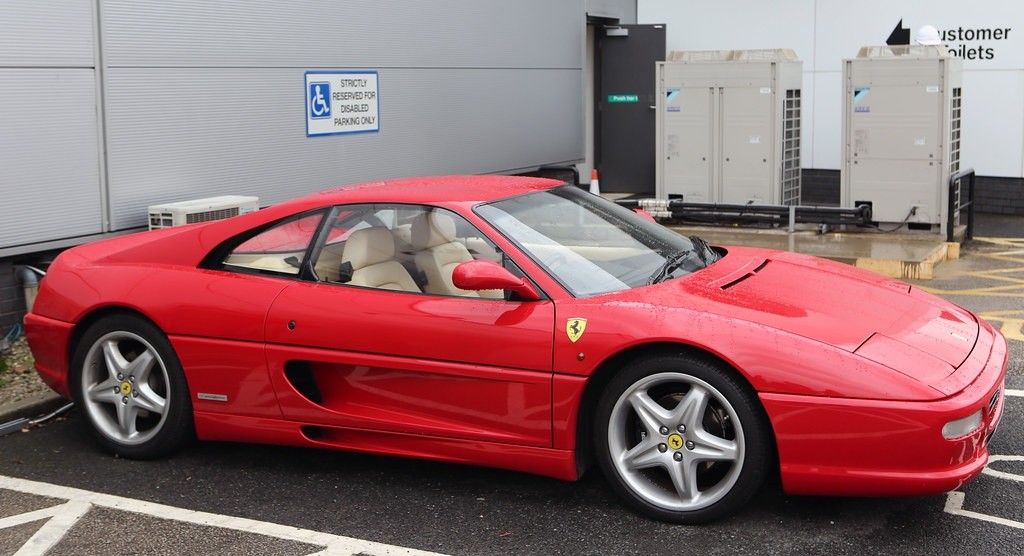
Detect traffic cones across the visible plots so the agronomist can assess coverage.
[589,169,601,197]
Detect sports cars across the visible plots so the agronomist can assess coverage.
[22,174,1009,528]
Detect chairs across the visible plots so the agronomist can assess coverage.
[342,225,424,294]
[410,213,504,298]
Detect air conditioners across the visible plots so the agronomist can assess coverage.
[148,195,259,232]
